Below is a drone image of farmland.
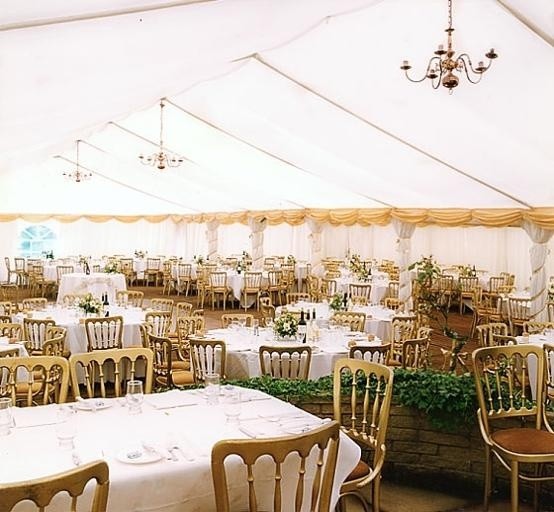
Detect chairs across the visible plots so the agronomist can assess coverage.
[469,285,497,337]
[240,272,262,313]
[0,348,20,385]
[4,257,23,288]
[1,356,70,407]
[28,259,41,290]
[490,323,507,345]
[189,338,226,384]
[151,298,174,313]
[0,459,110,512]
[388,320,415,365]
[85,316,124,396]
[15,327,66,406]
[33,266,56,297]
[476,325,494,372]
[492,334,529,388]
[64,294,85,301]
[509,298,531,336]
[438,275,465,314]
[484,293,509,324]
[148,333,193,387]
[178,264,196,297]
[69,348,154,400]
[398,337,428,371]
[116,291,128,306]
[281,270,294,292]
[286,293,311,304]
[145,311,172,337]
[334,358,394,511]
[417,267,440,293]
[349,283,371,305]
[260,297,275,327]
[268,271,283,306]
[385,297,405,314]
[523,322,554,336]
[458,277,479,315]
[140,322,190,370]
[212,420,341,512]
[1,323,23,341]
[162,260,176,296]
[488,276,504,293]
[500,272,509,285]
[14,258,28,285]
[380,282,400,308]
[53,266,74,301]
[93,264,100,272]
[306,274,322,302]
[222,313,254,328]
[196,270,211,308]
[1,284,18,313]
[176,317,205,358]
[472,288,496,337]
[321,277,337,301]
[193,310,205,317]
[120,258,138,287]
[128,290,144,307]
[23,318,71,360]
[22,298,48,308]
[350,342,392,365]
[497,286,516,294]
[143,258,160,288]
[176,302,193,317]
[412,326,434,367]
[259,345,312,381]
[472,344,553,512]
[209,272,234,311]
[333,311,366,333]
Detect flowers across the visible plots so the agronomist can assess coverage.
[274,313,299,338]
[78,293,102,313]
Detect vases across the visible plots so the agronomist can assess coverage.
[278,334,297,341]
[87,312,97,318]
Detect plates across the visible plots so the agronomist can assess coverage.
[74,398,114,410]
[114,445,166,464]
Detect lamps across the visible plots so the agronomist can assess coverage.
[139,100,183,170]
[62,141,93,182]
[400,0,498,97]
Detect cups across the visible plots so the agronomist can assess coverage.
[223,389,241,424]
[204,373,220,406]
[0,398,12,436]
[126,380,143,413]
